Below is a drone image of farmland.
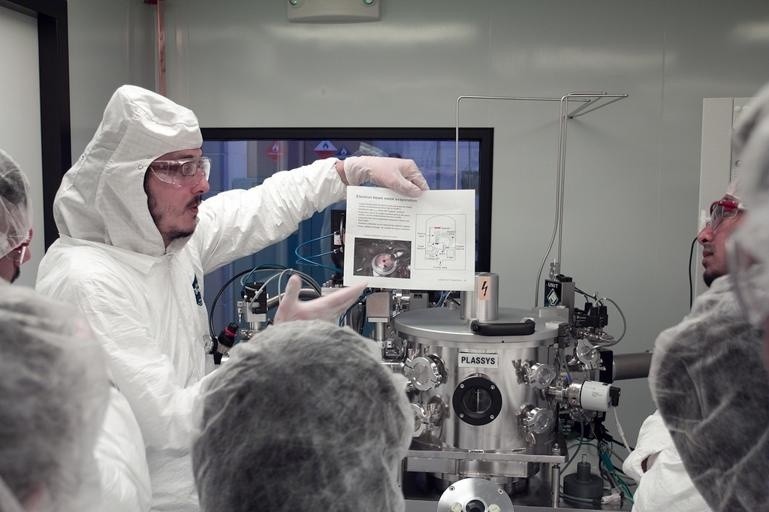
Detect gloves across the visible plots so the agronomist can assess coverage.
[344,153,430,197]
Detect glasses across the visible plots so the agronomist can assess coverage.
[149,153,213,188]
[703,199,744,233]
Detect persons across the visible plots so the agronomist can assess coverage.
[0,276,110,511]
[183,317,416,511]
[34,80,433,511]
[0,146,154,511]
[622,85,769,512]
